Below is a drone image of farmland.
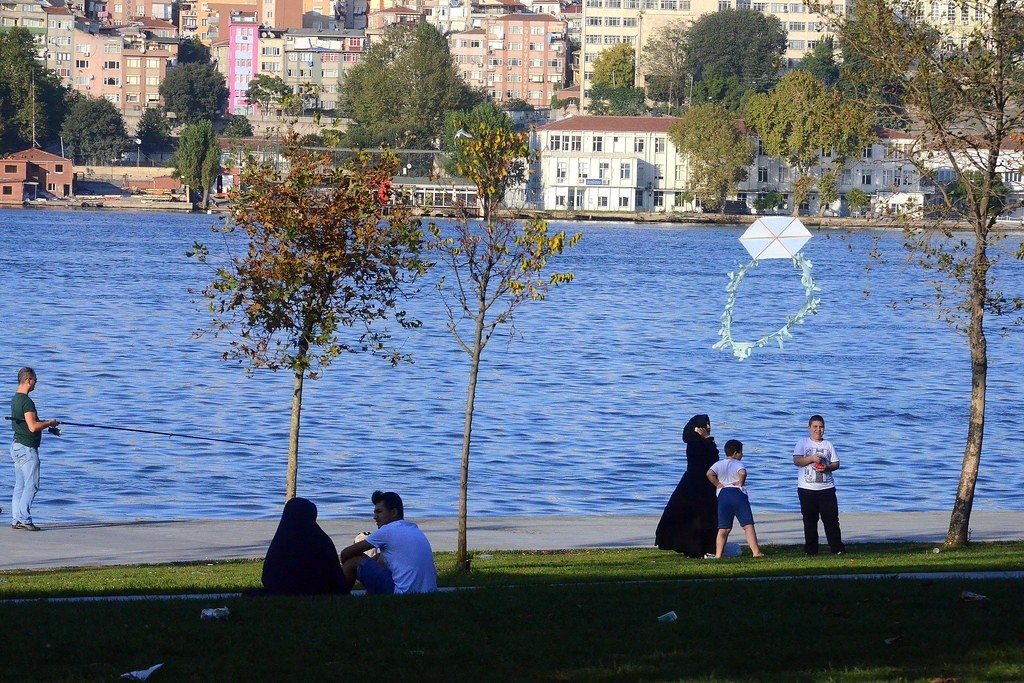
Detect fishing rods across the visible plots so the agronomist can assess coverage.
[4,415,319,455]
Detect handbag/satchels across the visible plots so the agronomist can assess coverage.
[354,531,378,557]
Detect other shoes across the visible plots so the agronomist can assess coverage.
[12,521,21,529]
[704,553,719,560]
[753,553,767,558]
[16,521,41,531]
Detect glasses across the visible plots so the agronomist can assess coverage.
[25,377,37,381]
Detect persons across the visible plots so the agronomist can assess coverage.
[706,440,765,559]
[792,414,846,555]
[653,414,721,559]
[339,492,437,594]
[9,365,59,531]
[260,497,352,599]
